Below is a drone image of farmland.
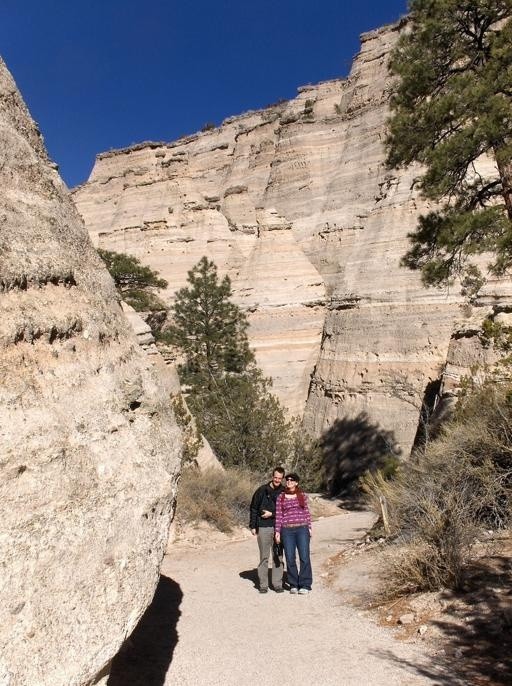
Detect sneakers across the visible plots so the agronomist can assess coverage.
[290,588,298,594]
[274,589,284,593]
[260,589,267,593]
[298,588,308,594]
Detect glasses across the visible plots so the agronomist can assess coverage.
[286,479,295,481]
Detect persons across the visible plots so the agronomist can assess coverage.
[274,473,313,594]
[249,467,285,593]
